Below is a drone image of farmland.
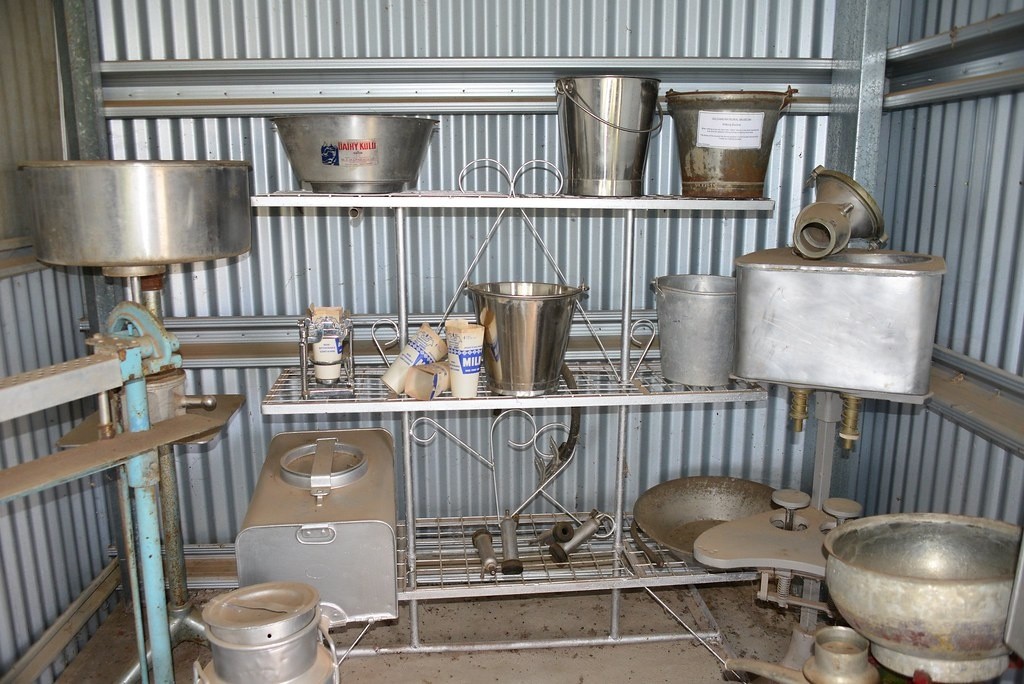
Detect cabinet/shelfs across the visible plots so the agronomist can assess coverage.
[250,189,775,602]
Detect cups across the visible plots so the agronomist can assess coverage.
[309,303,342,379]
[405,362,451,401]
[380,323,448,396]
[445,320,485,397]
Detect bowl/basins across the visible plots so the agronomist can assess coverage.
[266,114,440,194]
[823,513,1023,683]
[634,475,784,562]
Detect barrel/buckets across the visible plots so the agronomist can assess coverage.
[650,274,738,387]
[555,74,663,197]
[467,278,589,397]
[667,86,798,199]
